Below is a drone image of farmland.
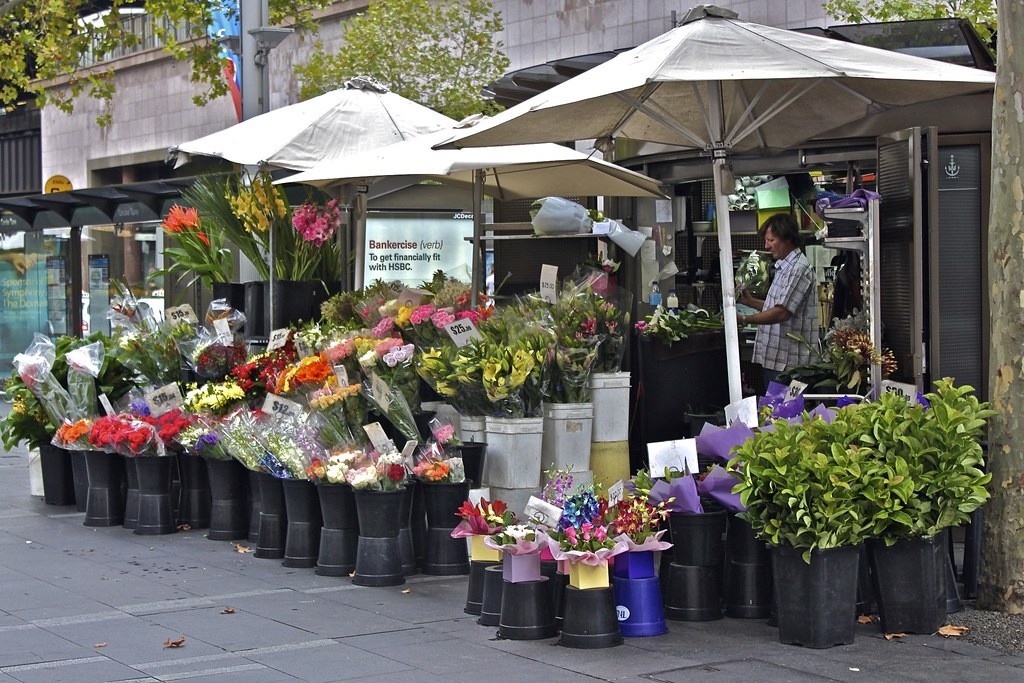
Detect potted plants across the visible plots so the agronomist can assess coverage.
[726,378,998,649]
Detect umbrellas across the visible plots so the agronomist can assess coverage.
[165,76,670,330]
[431,5,996,404]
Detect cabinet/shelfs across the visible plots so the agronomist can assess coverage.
[824,208,868,256]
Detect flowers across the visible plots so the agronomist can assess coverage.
[774,310,898,392]
[0,167,628,490]
[452,458,738,555]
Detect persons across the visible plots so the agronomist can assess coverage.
[720,213,818,396]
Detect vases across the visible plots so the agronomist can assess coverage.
[590,441,630,499]
[210,282,245,334]
[420,401,446,412]
[464,512,771,650]
[437,404,460,437]
[462,442,489,490]
[541,403,594,473]
[314,279,341,327]
[242,281,265,338]
[588,371,631,443]
[543,468,593,498]
[459,415,486,443]
[263,279,316,339]
[490,486,542,521]
[485,415,544,490]
[39,447,469,587]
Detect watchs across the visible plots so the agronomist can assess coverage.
[742,315,748,327]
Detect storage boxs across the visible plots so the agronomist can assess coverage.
[729,176,793,232]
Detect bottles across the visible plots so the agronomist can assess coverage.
[667,289,679,315]
[648,281,662,308]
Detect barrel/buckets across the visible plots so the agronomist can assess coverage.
[463,557,774,649]
[82,487,468,588]
